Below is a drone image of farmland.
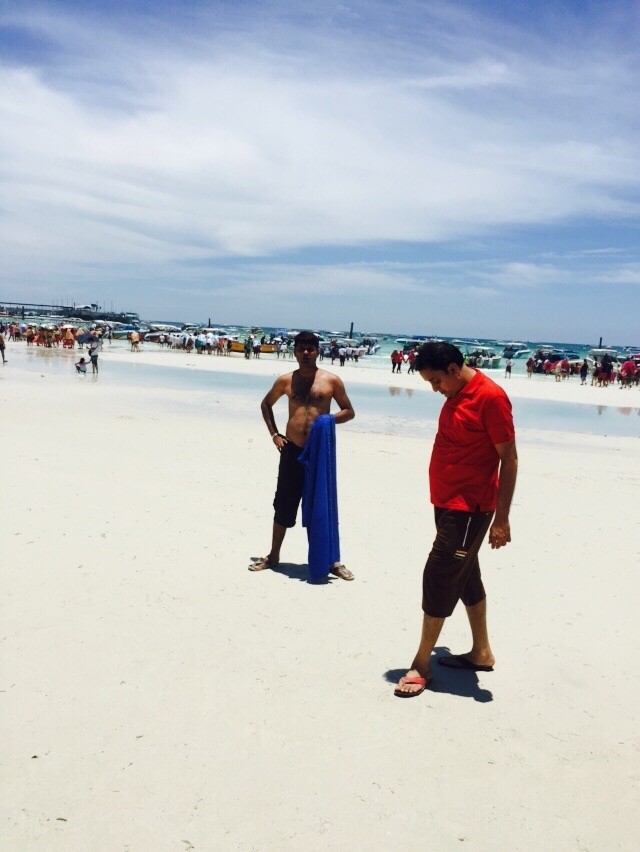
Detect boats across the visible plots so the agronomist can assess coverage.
[0,301,640,385]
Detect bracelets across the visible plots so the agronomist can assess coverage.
[271,433,280,440]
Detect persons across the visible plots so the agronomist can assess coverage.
[391,341,519,698]
[243,331,357,584]
[1,301,640,390]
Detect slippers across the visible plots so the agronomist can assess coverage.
[393,673,433,697]
[438,653,494,672]
[248,561,273,571]
[330,565,355,580]
